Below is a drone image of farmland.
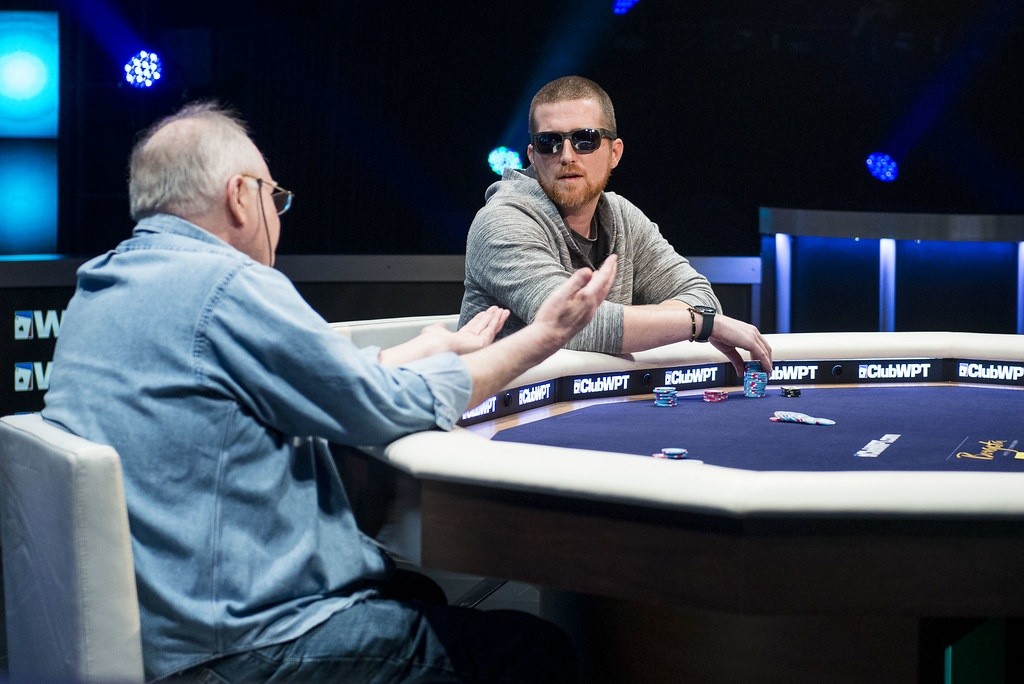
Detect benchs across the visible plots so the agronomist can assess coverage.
[0,314,459,684]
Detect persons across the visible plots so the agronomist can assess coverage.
[41,106,617,684]
[456,76,773,378]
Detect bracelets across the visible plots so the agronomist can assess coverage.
[686,308,696,342]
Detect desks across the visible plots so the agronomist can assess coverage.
[384,331,1023,684]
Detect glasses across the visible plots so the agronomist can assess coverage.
[241,173,294,215]
[531,127,616,156]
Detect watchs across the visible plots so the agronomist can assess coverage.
[692,306,716,343]
